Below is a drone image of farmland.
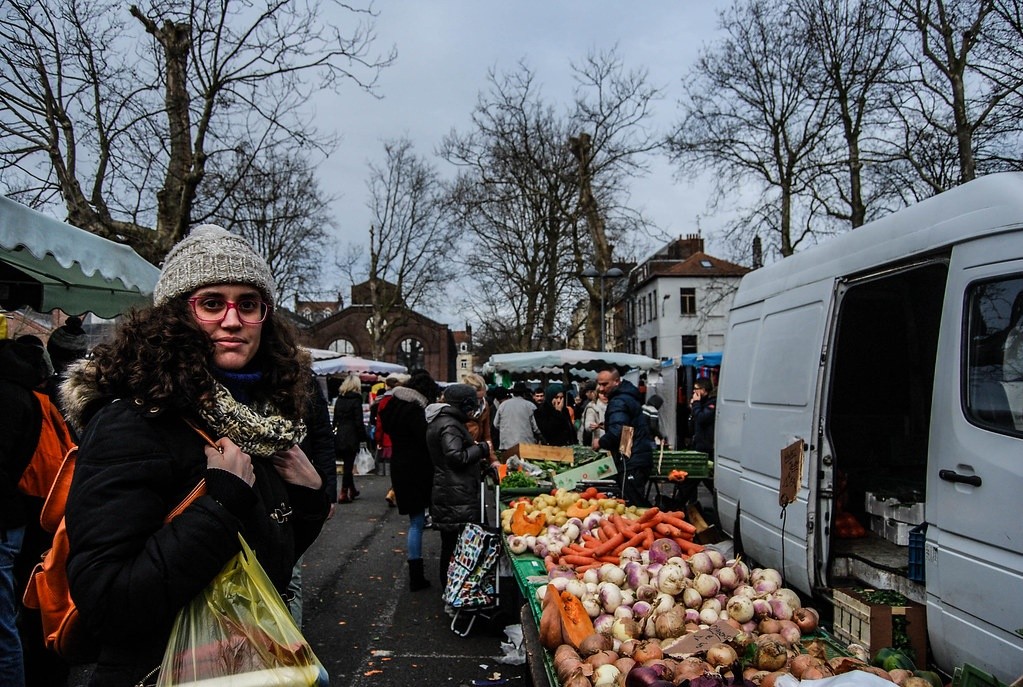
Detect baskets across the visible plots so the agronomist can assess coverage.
[650,449,709,479]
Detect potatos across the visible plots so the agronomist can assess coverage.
[501,489,647,532]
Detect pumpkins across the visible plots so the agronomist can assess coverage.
[510,503,545,536]
[540,584,594,648]
[565,501,599,519]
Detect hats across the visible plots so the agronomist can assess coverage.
[443,383,478,408]
[152,223,277,314]
[46,315,90,361]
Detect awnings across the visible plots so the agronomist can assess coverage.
[661,352,723,368]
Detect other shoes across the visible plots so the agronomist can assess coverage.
[385,491,398,508]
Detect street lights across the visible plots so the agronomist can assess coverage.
[580,267,626,353]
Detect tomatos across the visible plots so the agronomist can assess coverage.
[835,488,862,536]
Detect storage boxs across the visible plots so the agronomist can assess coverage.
[865,491,927,584]
[650,449,708,479]
[502,442,618,491]
[833,589,926,672]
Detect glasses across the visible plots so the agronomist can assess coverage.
[187,296,273,326]
[693,387,702,390]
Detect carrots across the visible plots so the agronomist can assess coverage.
[545,508,703,577]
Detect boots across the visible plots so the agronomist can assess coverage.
[338,488,350,504]
[407,558,431,593]
[347,485,360,501]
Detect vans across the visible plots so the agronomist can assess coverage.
[708,166,1023,687]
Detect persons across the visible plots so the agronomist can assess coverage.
[289,367,337,630]
[679,377,717,513]
[368,369,500,620]
[0,316,88,687]
[485,366,668,507]
[332,375,369,504]
[58,224,331,687]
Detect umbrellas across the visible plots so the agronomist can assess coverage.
[296,346,408,382]
[489,348,662,393]
[482,361,599,388]
[0,194,161,320]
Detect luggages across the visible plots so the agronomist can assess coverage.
[441,469,503,637]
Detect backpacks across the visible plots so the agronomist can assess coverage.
[16,389,79,500]
[20,405,229,669]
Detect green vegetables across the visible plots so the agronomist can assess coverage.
[501,445,606,488]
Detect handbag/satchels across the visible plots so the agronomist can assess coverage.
[154,531,329,687]
[351,447,376,476]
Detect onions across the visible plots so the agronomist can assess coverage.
[536,537,819,642]
[535,608,943,687]
[507,511,611,555]
